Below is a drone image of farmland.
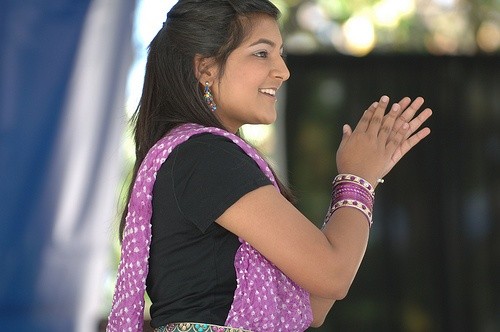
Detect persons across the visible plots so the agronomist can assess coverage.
[105,1,433,332]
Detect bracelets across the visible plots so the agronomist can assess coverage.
[325,174,376,228]
